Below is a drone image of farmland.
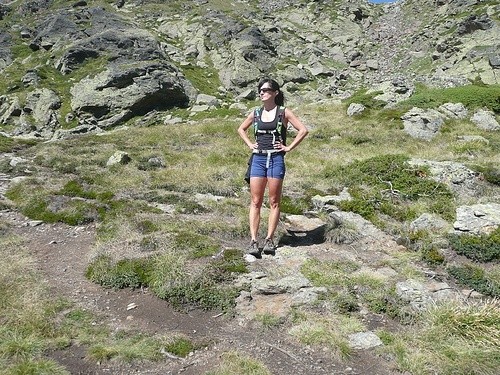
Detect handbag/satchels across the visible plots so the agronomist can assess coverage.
[244,152,254,183]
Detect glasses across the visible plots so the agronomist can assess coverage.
[258,88,275,94]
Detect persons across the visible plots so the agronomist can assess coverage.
[237,79,308,255]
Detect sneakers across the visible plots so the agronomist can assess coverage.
[249,239,261,258]
[263,237,277,253]
